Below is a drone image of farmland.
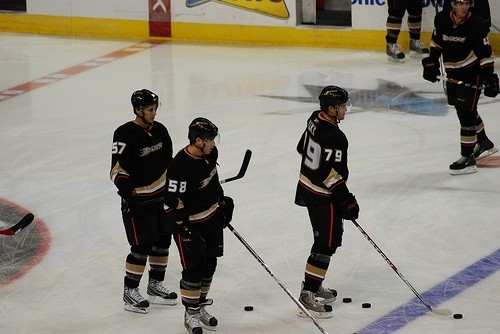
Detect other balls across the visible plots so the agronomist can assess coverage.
[244,306,253,312]
[362,303,372,309]
[453,313,463,320]
[342,297,352,304]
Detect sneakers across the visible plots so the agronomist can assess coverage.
[147,277,178,306]
[409,39,430,59]
[199,299,218,332]
[387,44,405,63]
[298,289,333,319]
[184,307,203,334]
[472,140,497,163]
[449,157,478,176]
[301,281,337,303]
[123,287,150,314]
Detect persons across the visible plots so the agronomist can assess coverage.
[422,0,500,176]
[385,0,430,63]
[161,116,234,334]
[109,89,178,313]
[294,86,360,319]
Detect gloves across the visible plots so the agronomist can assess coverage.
[340,194,359,220]
[178,224,193,241]
[422,57,441,83]
[118,190,141,216]
[219,197,235,228]
[483,74,500,98]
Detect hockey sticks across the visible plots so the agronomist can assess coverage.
[436,75,500,94]
[434,0,448,96]
[351,218,454,317]
[121,148,253,214]
[0,213,34,235]
[226,222,330,334]
[491,22,500,33]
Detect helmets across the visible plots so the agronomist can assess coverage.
[188,118,218,140]
[319,86,348,107]
[131,89,158,107]
[451,0,474,9]
